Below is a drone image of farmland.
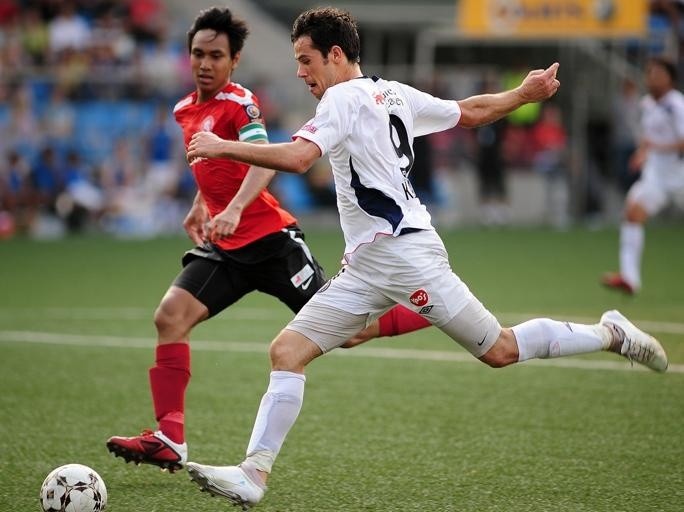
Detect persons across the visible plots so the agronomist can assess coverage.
[106,8,432,473]
[187,9,667,510]
[0,0,280,240]
[407,61,646,211]
[598,57,684,296]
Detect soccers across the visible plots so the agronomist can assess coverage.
[40,464,107,512]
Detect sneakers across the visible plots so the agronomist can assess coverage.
[599,309,668,371]
[184,462,265,511]
[106,429,188,474]
[601,273,634,294]
